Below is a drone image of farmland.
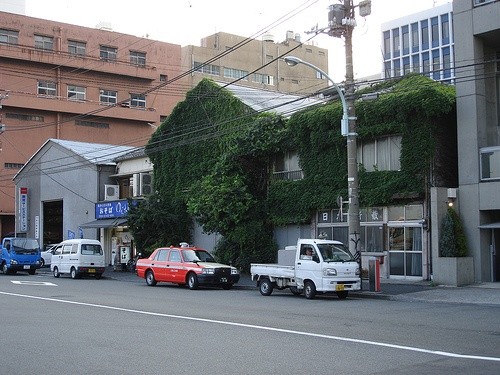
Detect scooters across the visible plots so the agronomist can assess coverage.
[126,251,147,272]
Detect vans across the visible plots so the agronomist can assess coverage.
[50,238,106,280]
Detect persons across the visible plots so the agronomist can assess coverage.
[303,247,318,261]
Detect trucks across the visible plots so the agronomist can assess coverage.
[250,237,362,299]
[0,237,42,275]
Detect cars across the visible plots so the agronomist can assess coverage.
[40,244,59,268]
[136,242,240,290]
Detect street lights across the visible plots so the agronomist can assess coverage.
[285,55,363,291]
